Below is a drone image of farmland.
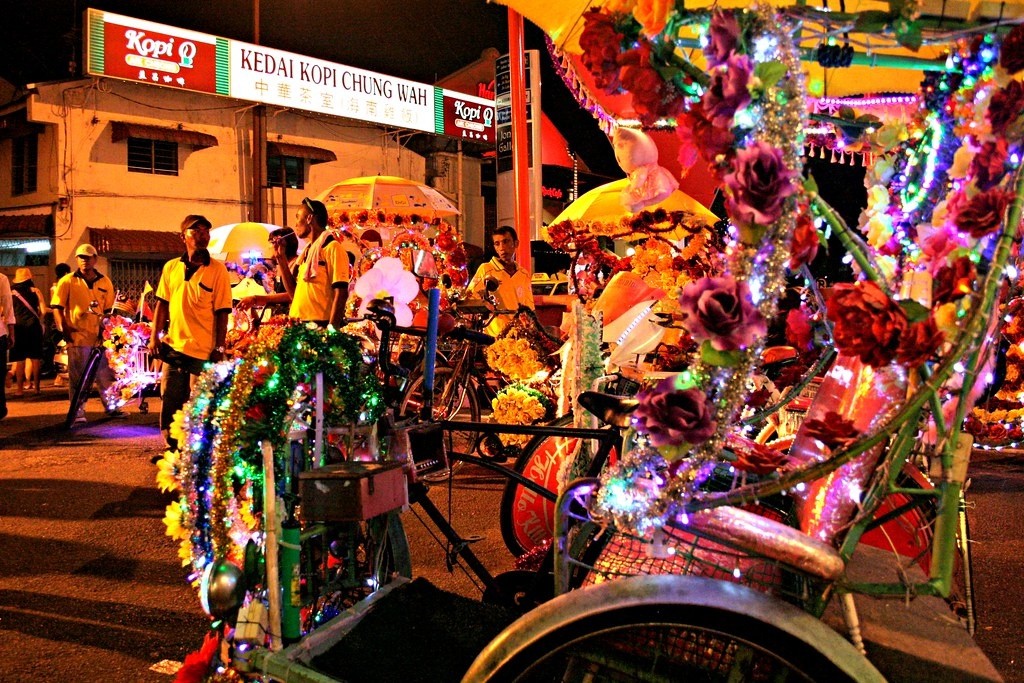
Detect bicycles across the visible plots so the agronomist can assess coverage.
[185,280,962,683]
[63,299,154,431]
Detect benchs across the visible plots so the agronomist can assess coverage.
[574,345,904,683]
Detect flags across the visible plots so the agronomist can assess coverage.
[112,292,136,315]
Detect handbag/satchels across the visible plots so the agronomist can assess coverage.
[40,321,45,334]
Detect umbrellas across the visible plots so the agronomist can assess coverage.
[311,175,461,222]
[208,222,307,284]
[546,176,722,243]
[491,0,1024,165]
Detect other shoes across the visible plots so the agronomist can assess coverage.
[0,407,7,419]
[483,435,507,462]
[24,383,34,389]
[149,454,164,465]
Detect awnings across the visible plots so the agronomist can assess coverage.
[89,228,188,253]
[0,214,53,238]
[267,141,337,165]
[110,120,219,153]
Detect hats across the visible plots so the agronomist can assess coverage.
[12,268,34,283]
[74,243,98,258]
[180,215,212,236]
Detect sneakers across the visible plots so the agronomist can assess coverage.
[105,409,131,417]
[75,408,86,422]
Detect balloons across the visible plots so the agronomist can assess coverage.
[611,124,679,213]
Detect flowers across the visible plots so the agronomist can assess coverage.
[539,0,1024,480]
[153,399,264,602]
[482,328,553,455]
[329,201,471,312]
[97,316,157,415]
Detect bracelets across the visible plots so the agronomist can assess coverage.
[214,346,225,353]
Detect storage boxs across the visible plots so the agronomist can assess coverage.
[300,459,409,524]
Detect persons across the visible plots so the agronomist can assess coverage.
[50,243,116,424]
[0,273,16,420]
[146,215,233,467]
[50,263,70,300]
[6,357,34,389]
[10,268,45,397]
[460,224,536,463]
[237,197,352,331]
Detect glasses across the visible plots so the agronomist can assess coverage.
[302,197,315,214]
